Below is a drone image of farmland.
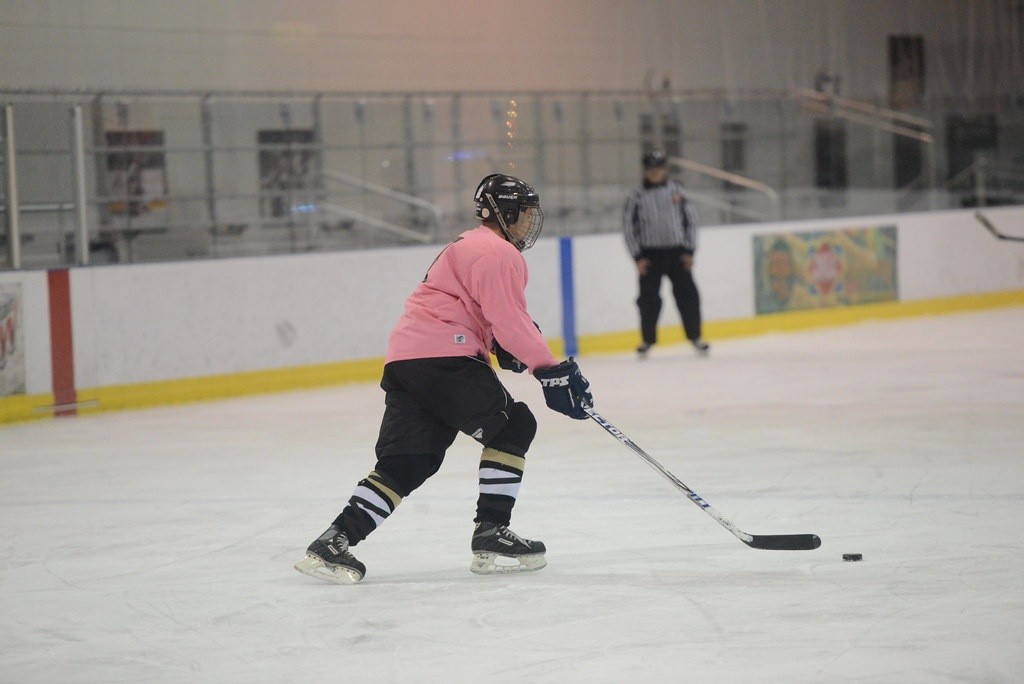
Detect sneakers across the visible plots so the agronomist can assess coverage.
[295,524,367,585]
[469,518,547,574]
[637,343,655,357]
[691,338,710,355]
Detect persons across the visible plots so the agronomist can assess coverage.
[294,173,593,587]
[621,148,712,360]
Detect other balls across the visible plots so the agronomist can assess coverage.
[842,552,863,562]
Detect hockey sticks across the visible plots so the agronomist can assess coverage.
[576,397,823,554]
[970,208,1024,249]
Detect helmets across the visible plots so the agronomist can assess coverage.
[640,149,671,168]
[473,173,539,225]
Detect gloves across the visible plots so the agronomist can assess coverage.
[533,356,593,420]
[492,320,541,373]
